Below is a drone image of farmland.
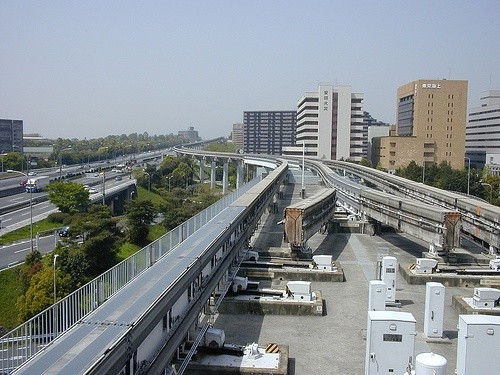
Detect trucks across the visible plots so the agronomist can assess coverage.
[24,176,50,193]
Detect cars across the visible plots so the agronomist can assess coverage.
[20,179,27,185]
[56,150,152,194]
[58,226,72,237]
[27,171,37,176]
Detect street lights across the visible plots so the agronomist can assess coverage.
[52,254,60,337]
[6,169,35,253]
[463,156,470,195]
